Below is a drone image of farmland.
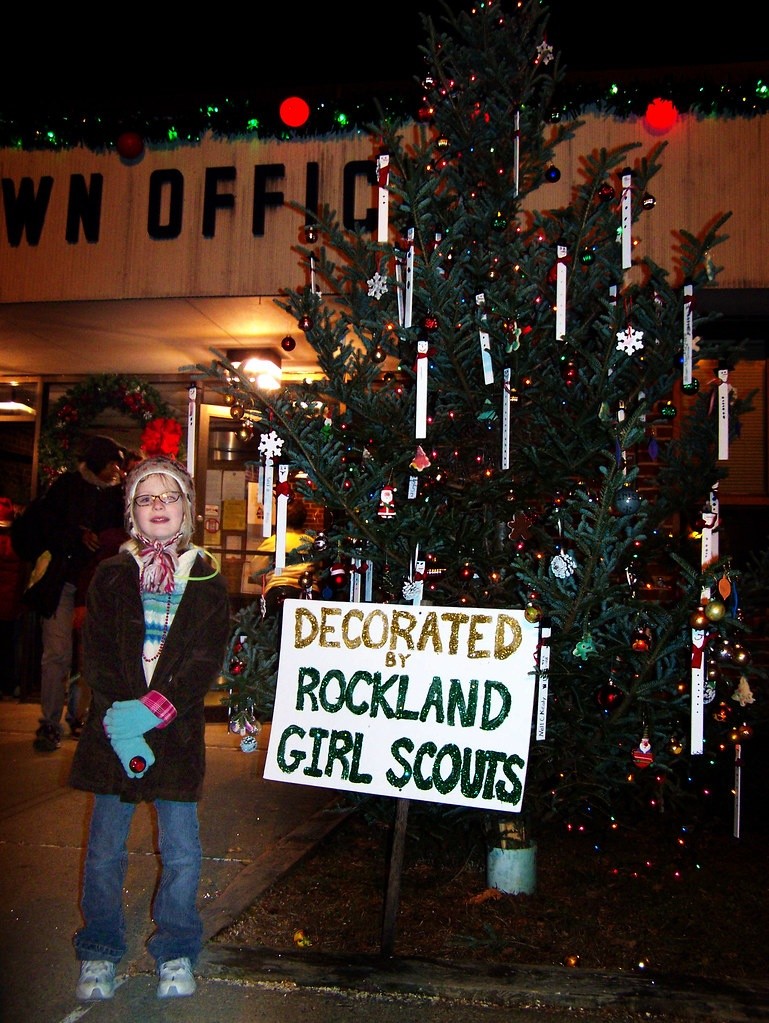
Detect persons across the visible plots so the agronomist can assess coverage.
[69,456,231,1000]
[13,436,125,752]
[250,498,324,618]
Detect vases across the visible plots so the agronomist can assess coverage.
[487,840,538,895]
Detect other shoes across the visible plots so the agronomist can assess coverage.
[33,725,61,752]
[69,720,84,740]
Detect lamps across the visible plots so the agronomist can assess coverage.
[225,347,283,391]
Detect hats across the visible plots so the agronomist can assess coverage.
[77,433,132,460]
[125,454,197,591]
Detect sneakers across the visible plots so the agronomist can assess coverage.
[75,960,116,1004]
[155,954,195,998]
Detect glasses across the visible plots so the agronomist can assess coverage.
[133,491,183,507]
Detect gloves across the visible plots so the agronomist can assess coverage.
[110,735,156,779]
[103,700,162,743]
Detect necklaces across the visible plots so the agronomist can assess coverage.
[139,561,170,662]
